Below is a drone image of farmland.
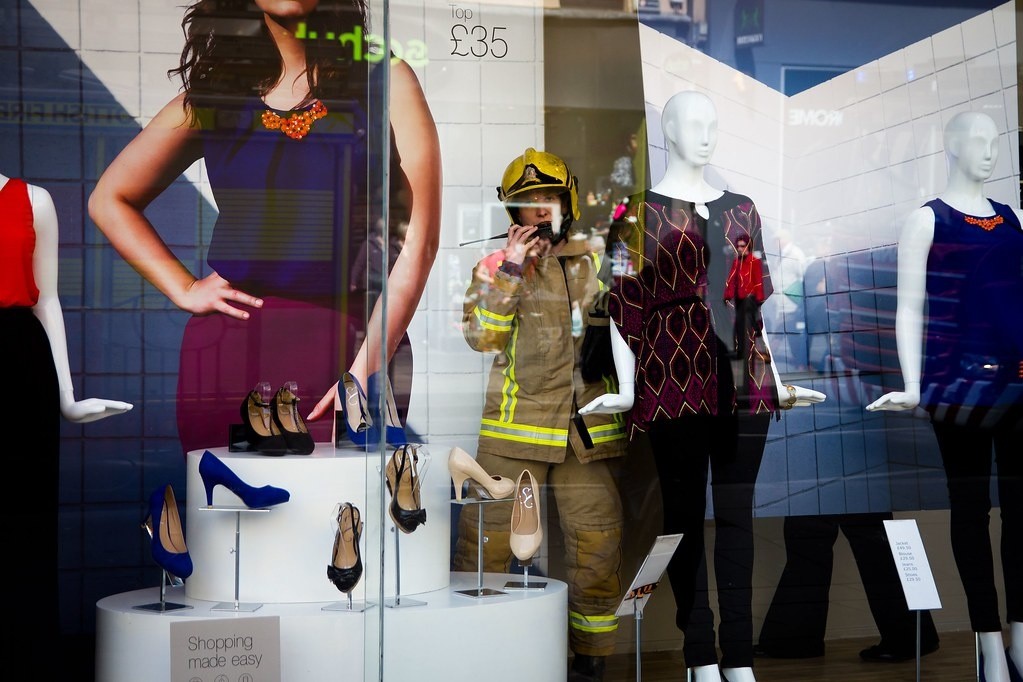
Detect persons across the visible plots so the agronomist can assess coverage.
[864,112,1023,682]
[577,90,826,682]
[751,511,940,664]
[0,168,132,654]
[460,150,631,663]
[82,2,439,457]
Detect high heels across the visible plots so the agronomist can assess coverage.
[367,372,407,448]
[199,450,290,509]
[240,390,286,456]
[143,483,193,578]
[328,503,363,593]
[448,447,515,502]
[386,445,426,534]
[270,387,314,454]
[338,372,378,452]
[509,469,543,561]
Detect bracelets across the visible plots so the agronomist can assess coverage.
[779,381,797,410]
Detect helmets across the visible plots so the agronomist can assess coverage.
[496,148,580,243]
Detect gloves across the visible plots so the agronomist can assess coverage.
[579,309,615,382]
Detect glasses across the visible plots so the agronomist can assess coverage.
[739,245,744,247]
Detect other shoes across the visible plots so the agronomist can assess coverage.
[859,641,940,663]
[752,644,768,658]
